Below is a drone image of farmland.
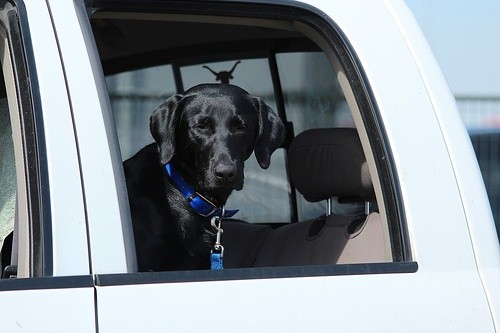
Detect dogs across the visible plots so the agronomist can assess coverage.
[0,83,286,280]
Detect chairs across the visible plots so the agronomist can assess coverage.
[252,128,391,268]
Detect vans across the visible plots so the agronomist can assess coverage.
[0,0,500,333]
